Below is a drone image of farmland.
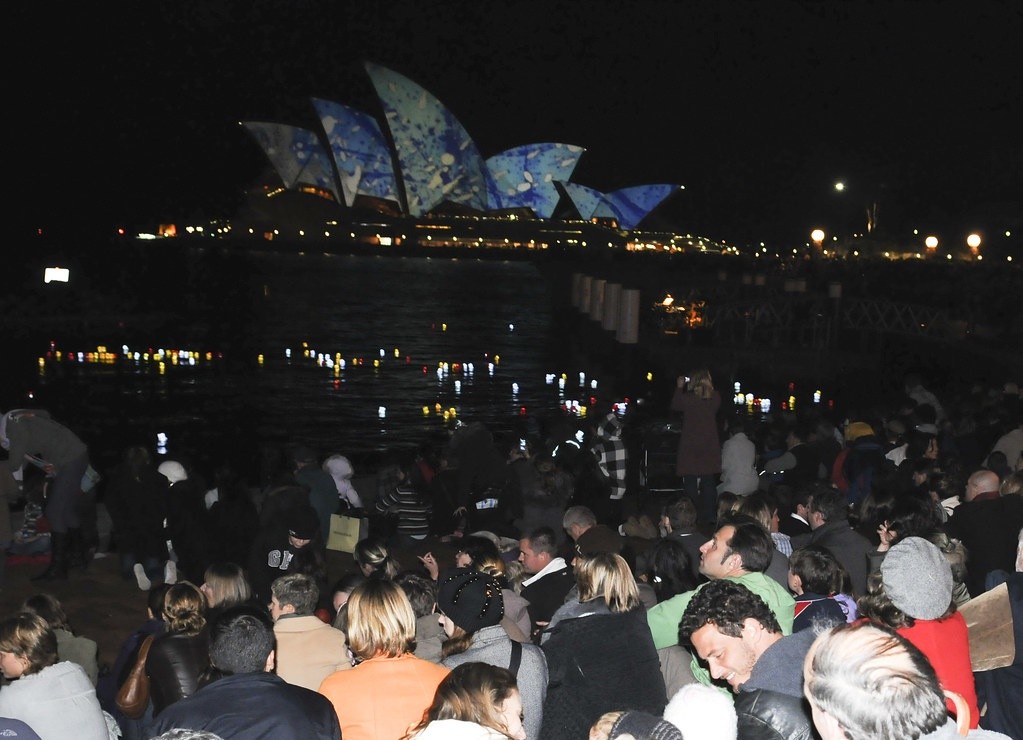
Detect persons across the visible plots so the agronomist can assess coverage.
[0,364,1023,740]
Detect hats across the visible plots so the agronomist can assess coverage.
[878,537,952,619]
[437,571,503,632]
[293,447,315,466]
[500,586,533,645]
[573,523,622,561]
[461,534,499,563]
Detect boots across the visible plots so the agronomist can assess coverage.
[68,537,88,568]
[35,549,65,581]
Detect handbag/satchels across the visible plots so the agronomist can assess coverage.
[113,631,160,720]
